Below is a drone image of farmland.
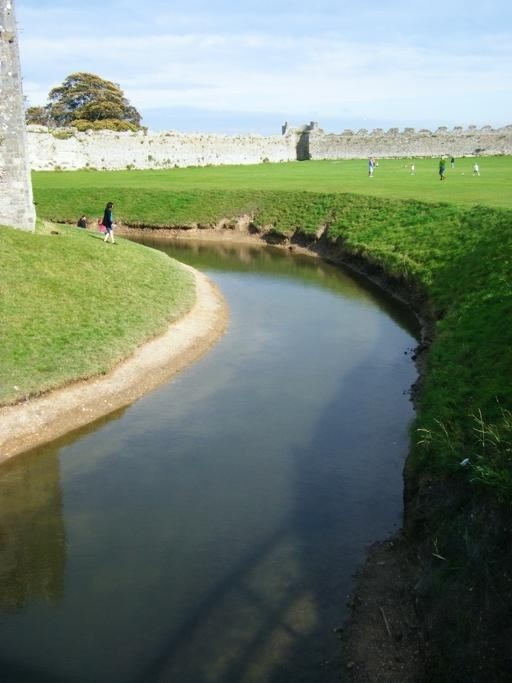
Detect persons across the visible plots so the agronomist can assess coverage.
[103,201,118,243]
[439,157,447,179]
[78,214,87,227]
[98,214,107,234]
[368,156,374,177]
[450,155,454,167]
[410,163,415,175]
[472,162,480,176]
